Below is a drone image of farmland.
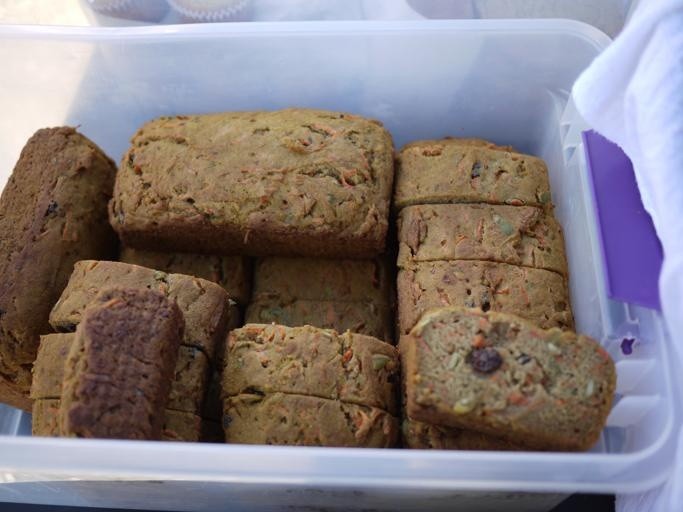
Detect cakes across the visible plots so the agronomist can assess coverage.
[170,0,255,23]
[96,238,248,339]
[107,106,400,264]
[395,203,567,278]
[393,259,576,335]
[217,388,404,450]
[86,0,168,22]
[61,285,186,440]
[31,399,211,443]
[402,416,526,453]
[28,333,215,426]
[48,260,229,366]
[405,304,618,451]
[241,298,397,347]
[250,253,395,306]
[392,135,554,211]
[216,322,401,414]
[0,124,117,415]
